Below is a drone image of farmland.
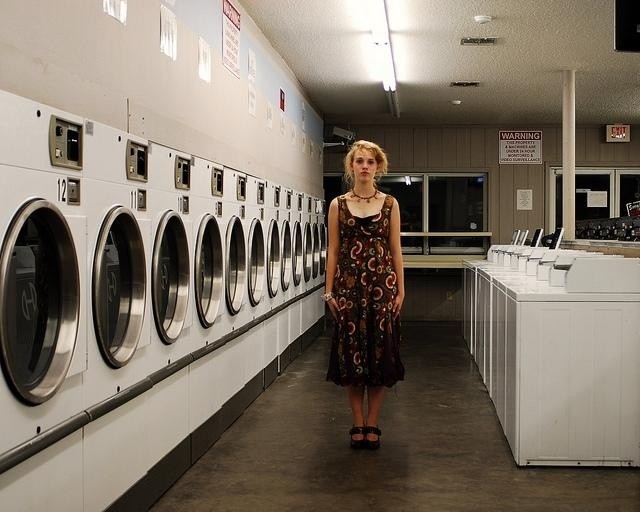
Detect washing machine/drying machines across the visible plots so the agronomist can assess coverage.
[466,228,639,467]
[1,89,325,511]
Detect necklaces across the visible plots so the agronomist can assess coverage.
[349,188,380,203]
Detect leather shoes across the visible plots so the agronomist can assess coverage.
[349,424,365,449]
[366,424,381,450]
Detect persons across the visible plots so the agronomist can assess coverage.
[321,139,407,450]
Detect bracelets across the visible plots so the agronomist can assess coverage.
[321,292,334,302]
